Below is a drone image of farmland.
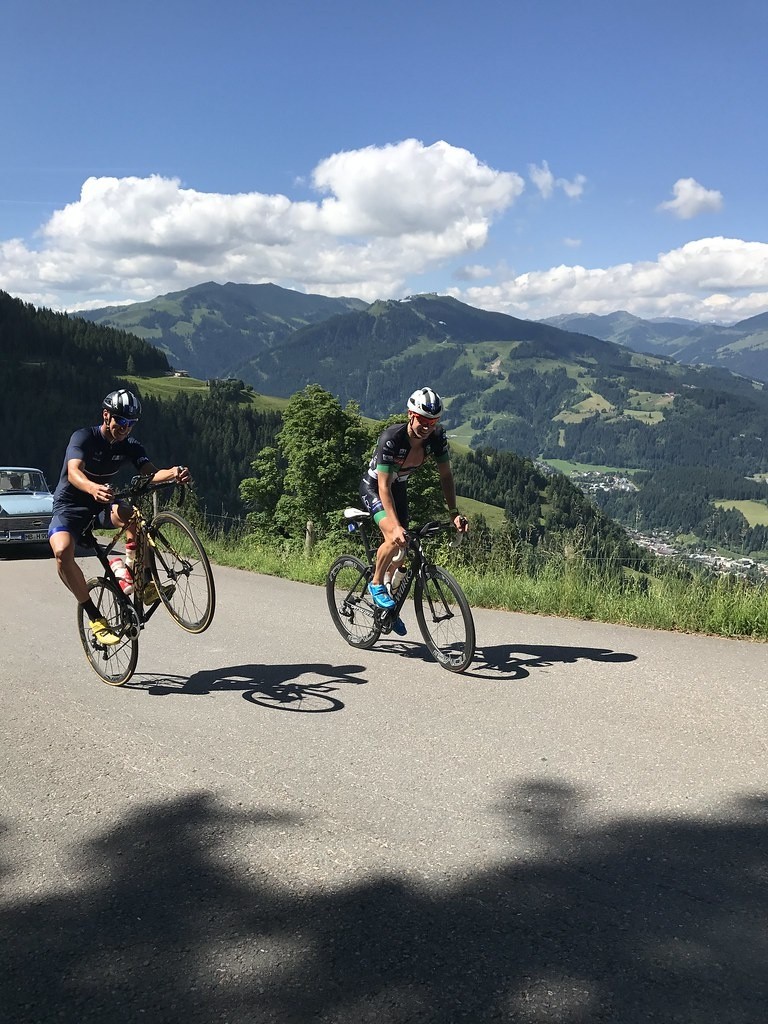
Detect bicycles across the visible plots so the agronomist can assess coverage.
[326,508,477,676]
[76,466,217,687]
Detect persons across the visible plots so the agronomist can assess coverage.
[10,475,21,489]
[48,389,192,646]
[359,386,469,636]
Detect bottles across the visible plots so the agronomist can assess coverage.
[125,542,136,570]
[109,557,134,595]
[383,572,393,599]
[390,567,407,595]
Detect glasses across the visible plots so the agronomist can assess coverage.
[113,416,138,427]
[412,413,439,425]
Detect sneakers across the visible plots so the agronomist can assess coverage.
[143,583,176,605]
[89,618,121,645]
[367,581,395,609]
[392,617,407,636]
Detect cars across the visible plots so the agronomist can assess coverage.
[0,466,55,548]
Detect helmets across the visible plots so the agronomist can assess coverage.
[406,386,444,418]
[102,389,142,421]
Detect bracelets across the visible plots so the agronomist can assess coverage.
[449,508,458,515]
